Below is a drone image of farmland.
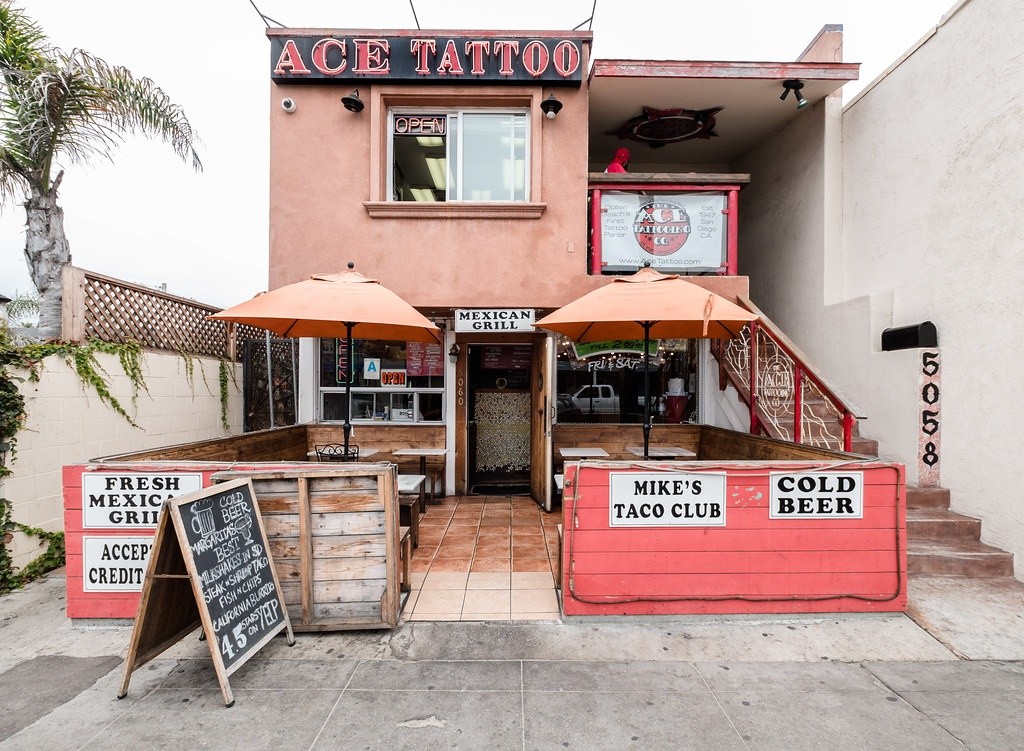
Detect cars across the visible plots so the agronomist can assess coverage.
[556,395,581,423]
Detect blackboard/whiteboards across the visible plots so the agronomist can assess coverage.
[168,477,290,678]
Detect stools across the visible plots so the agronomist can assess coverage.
[400,526,411,591]
[398,493,420,548]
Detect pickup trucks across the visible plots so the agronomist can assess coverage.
[570,384,665,419]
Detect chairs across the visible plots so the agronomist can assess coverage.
[314,442,359,462]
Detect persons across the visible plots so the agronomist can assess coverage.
[603,149,630,174]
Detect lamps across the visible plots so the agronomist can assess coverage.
[540,93,563,119]
[341,88,364,111]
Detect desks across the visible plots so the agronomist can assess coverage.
[626,446,696,461]
[391,448,449,513]
[554,474,563,488]
[559,447,610,461]
[307,446,379,461]
[398,474,426,491]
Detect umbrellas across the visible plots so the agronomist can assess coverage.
[205,269,440,461]
[533,262,761,459]
